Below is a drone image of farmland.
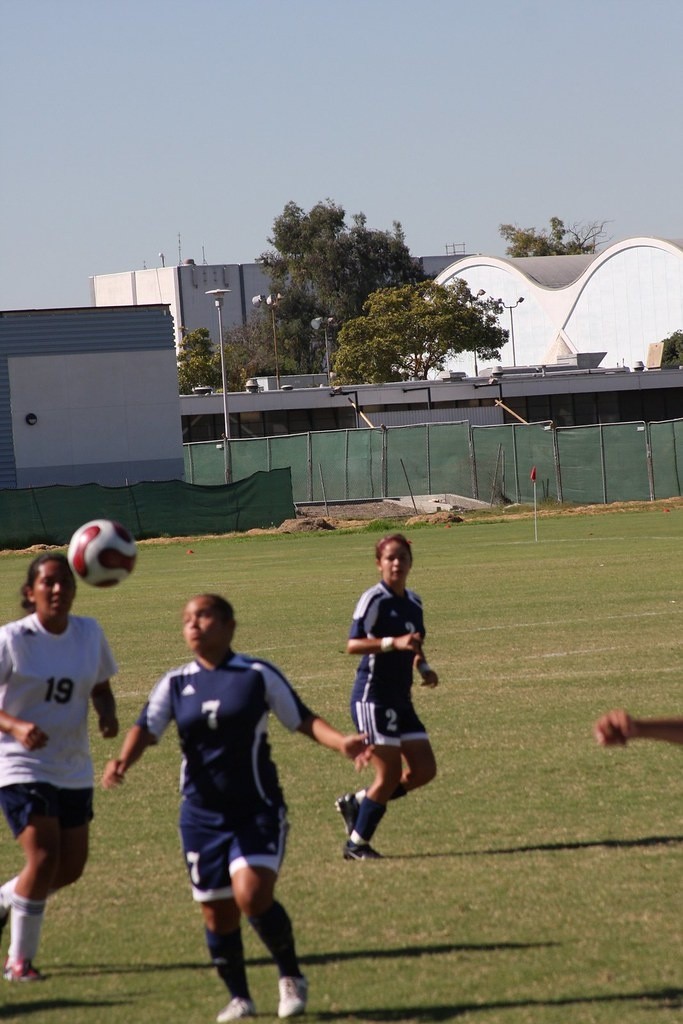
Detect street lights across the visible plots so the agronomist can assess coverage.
[470,288,485,376]
[310,316,333,389]
[492,297,525,366]
[205,287,235,485]
[250,293,281,391]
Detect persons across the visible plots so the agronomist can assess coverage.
[0,554,120,983]
[335,534,439,861]
[591,711,683,747]
[102,594,374,1021]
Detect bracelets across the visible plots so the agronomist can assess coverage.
[418,663,430,674]
[381,637,393,652]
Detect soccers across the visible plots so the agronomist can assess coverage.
[69,519,138,590]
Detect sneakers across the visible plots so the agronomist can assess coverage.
[335,793,360,836]
[2,960,45,984]
[343,844,381,860]
[217,997,255,1023]
[278,974,308,1018]
